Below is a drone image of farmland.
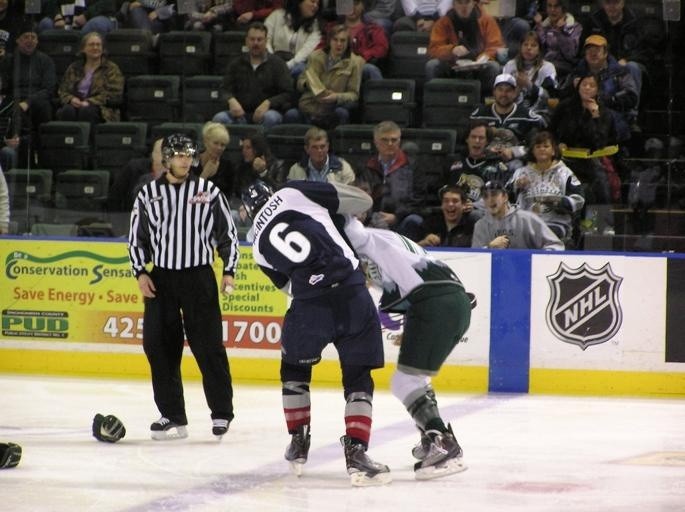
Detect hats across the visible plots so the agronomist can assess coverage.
[584,35,607,45]
[479,180,507,195]
[494,74,518,89]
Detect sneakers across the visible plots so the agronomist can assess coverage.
[150,417,188,430]
[212,419,228,436]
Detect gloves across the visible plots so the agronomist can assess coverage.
[92,413,125,443]
[1,441,21,468]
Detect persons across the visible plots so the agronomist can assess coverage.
[0,0,662,252]
[128,132,240,436]
[343,214,471,472]
[240,178,389,478]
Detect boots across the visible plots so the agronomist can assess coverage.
[285,424,311,463]
[412,422,461,472]
[340,434,391,474]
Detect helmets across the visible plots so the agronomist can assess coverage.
[241,179,272,219]
[161,133,199,171]
